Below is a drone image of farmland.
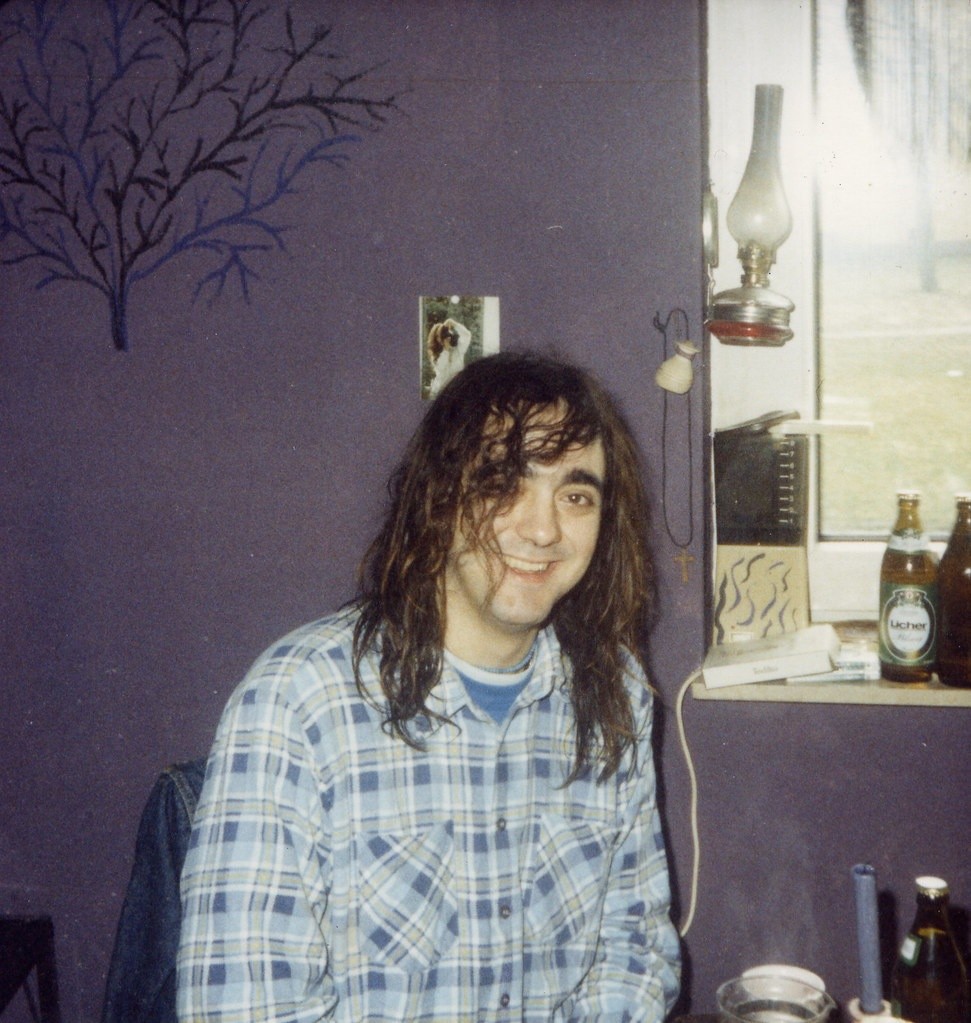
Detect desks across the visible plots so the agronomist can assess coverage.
[671,625,971,1023]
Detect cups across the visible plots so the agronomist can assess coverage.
[717,964,837,1023]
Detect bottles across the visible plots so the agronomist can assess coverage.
[878,489,971,690]
[894,876,971,1023]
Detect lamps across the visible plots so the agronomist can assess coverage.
[705,82,799,353]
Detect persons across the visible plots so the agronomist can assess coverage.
[174,355,682,1023]
[426,318,471,401]
